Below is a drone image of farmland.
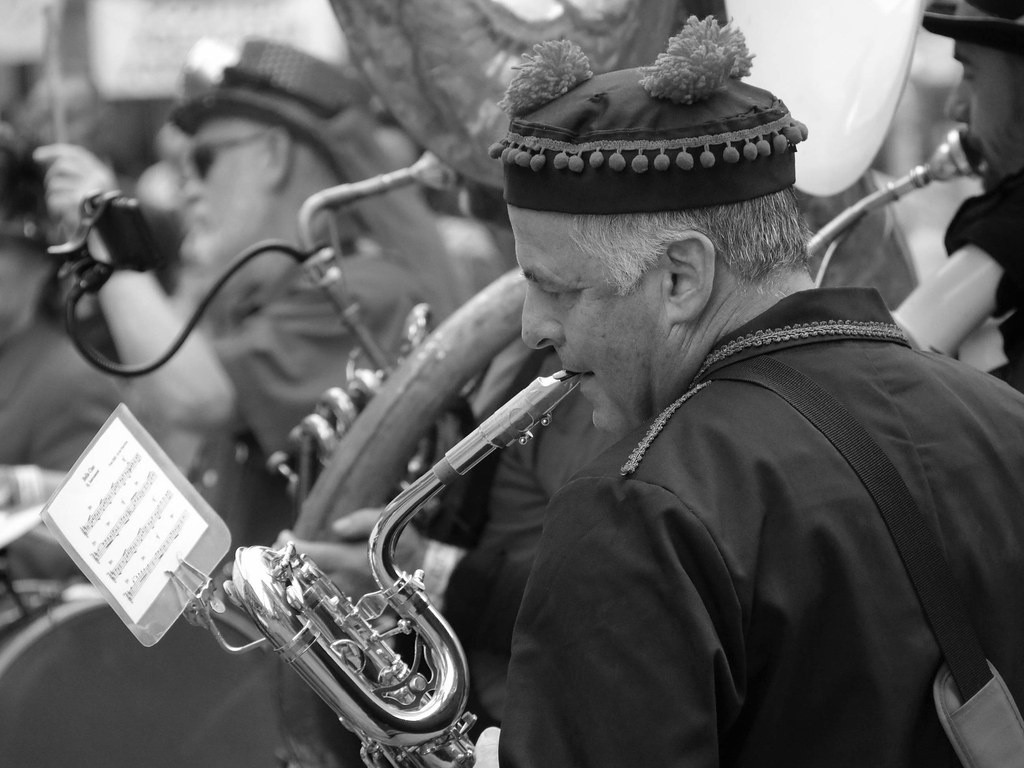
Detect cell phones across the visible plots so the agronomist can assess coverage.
[87,198,158,273]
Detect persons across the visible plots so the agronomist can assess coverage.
[2,125,134,507]
[29,35,380,469]
[486,16,1024,768]
[922,1,1023,394]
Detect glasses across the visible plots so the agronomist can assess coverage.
[190,130,290,180]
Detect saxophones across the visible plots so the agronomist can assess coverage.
[262,148,484,548]
[802,125,990,292]
[220,364,589,768]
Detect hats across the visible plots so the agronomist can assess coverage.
[487,15,808,215]
[173,35,378,172]
[921,0,1024,50]
